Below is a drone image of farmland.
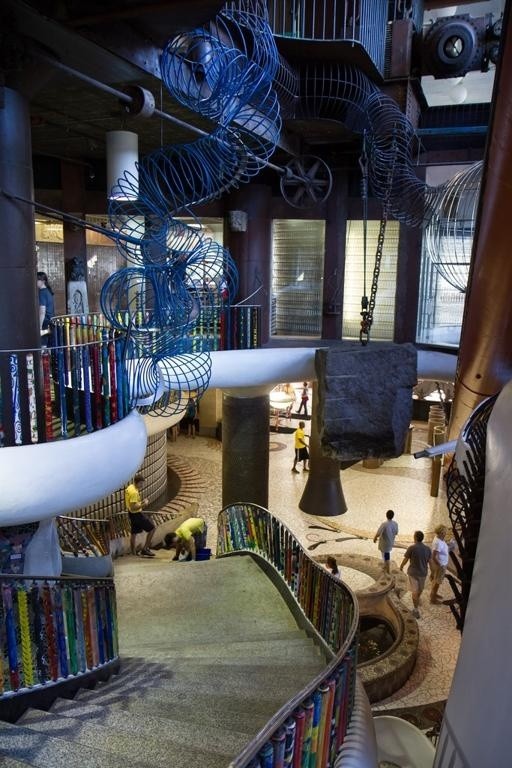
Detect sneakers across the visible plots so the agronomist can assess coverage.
[430,592,443,605]
[303,468,309,471]
[141,547,155,556]
[413,609,420,618]
[292,468,299,473]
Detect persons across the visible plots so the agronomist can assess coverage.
[72,289,84,313]
[187,398,197,439]
[373,510,449,619]
[164,517,207,562]
[36,272,56,351]
[283,382,309,416]
[124,474,156,557]
[291,421,311,473]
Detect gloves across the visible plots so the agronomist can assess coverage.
[143,498,150,506]
